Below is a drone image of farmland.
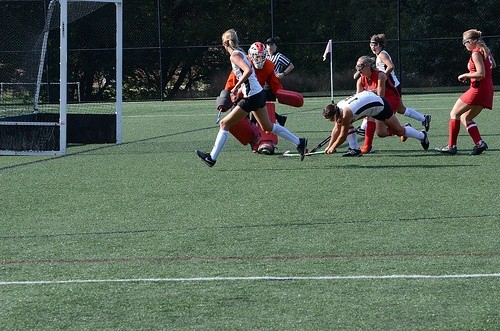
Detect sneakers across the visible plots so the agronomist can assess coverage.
[296,138,309,161]
[401,123,412,142]
[434,146,458,156]
[421,114,432,132]
[419,130,430,151]
[196,150,216,168]
[258,141,275,155]
[354,126,366,136]
[468,140,489,156]
[343,148,363,157]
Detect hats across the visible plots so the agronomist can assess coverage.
[266,38,279,47]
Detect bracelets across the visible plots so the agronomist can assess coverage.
[282,71,287,76]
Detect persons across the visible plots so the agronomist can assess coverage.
[353,33,431,143]
[217,37,294,155]
[194,28,308,168]
[434,28,497,156]
[323,90,429,155]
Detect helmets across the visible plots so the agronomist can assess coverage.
[248,41,267,69]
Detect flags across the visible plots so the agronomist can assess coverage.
[323,40,330,62]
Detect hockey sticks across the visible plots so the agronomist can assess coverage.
[282,149,337,156]
[309,134,333,152]
[216,108,222,124]
